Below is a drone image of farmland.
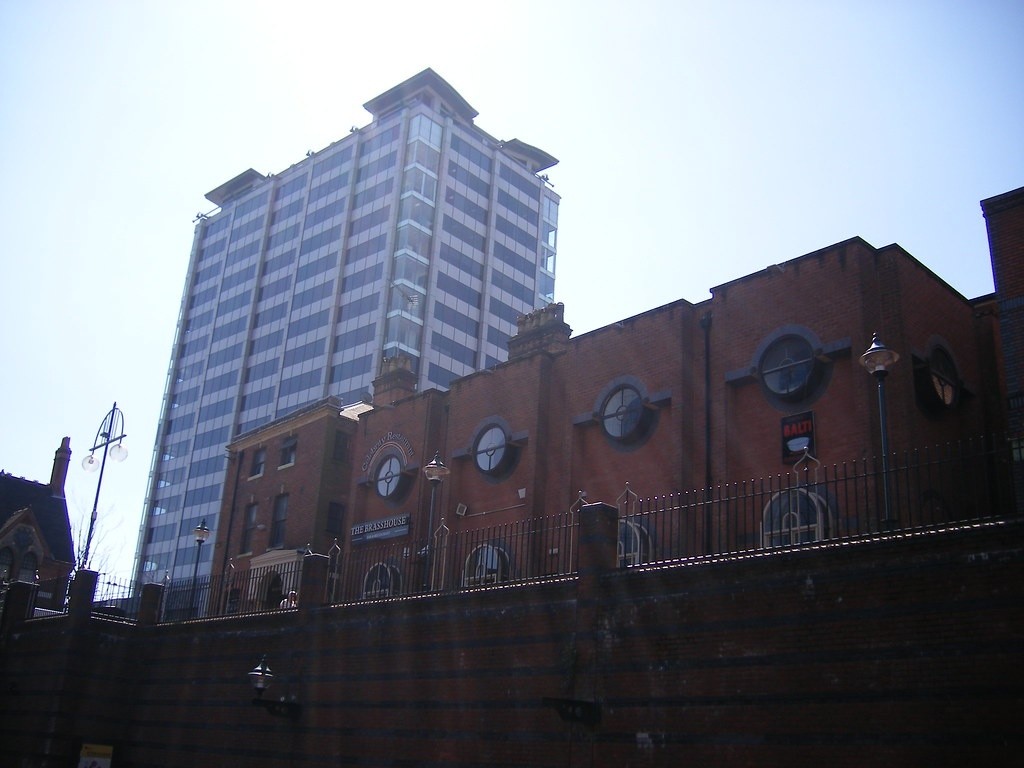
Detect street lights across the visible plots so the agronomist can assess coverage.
[81,400,128,570]
[421,449,451,592]
[859,331,902,530]
[184,517,212,619]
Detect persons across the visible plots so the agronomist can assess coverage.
[280,591,298,609]
